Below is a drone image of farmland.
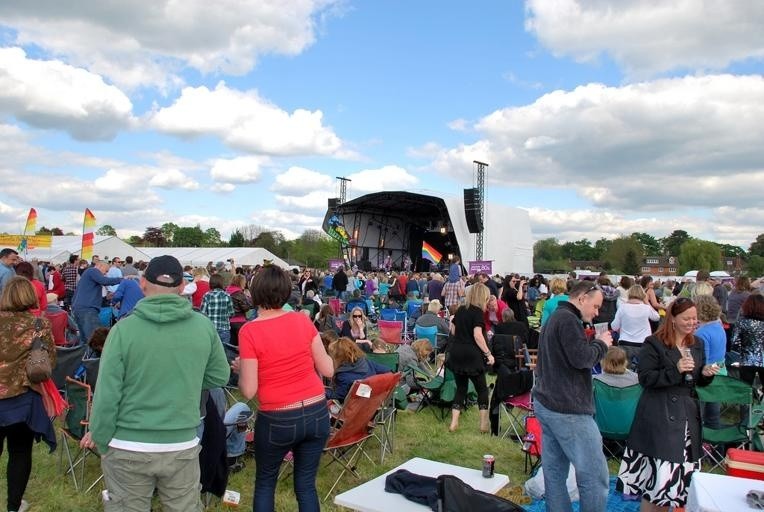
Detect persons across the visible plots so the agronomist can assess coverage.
[2,247,531,512]
[519,270,764,511]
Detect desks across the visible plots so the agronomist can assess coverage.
[331,455,509,512]
[684,471,764,510]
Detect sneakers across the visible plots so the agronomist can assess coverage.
[16,499,28,512]
[228,459,244,473]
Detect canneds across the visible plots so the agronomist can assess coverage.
[482,456,497,479]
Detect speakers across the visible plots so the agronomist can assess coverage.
[358,261,371,272]
[464,188,483,233]
[327,198,341,215]
[416,261,430,272]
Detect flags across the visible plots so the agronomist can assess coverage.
[422,240,442,266]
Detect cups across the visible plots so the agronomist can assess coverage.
[594,322,609,338]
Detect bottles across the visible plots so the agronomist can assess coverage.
[522,431,535,451]
[684,348,695,387]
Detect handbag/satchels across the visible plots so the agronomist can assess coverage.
[25,317,52,383]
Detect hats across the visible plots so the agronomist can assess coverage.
[45,292,57,303]
[144,255,183,287]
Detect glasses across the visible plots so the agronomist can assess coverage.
[353,315,361,318]
[578,283,600,301]
[674,297,691,305]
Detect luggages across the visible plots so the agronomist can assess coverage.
[724,447,764,479]
